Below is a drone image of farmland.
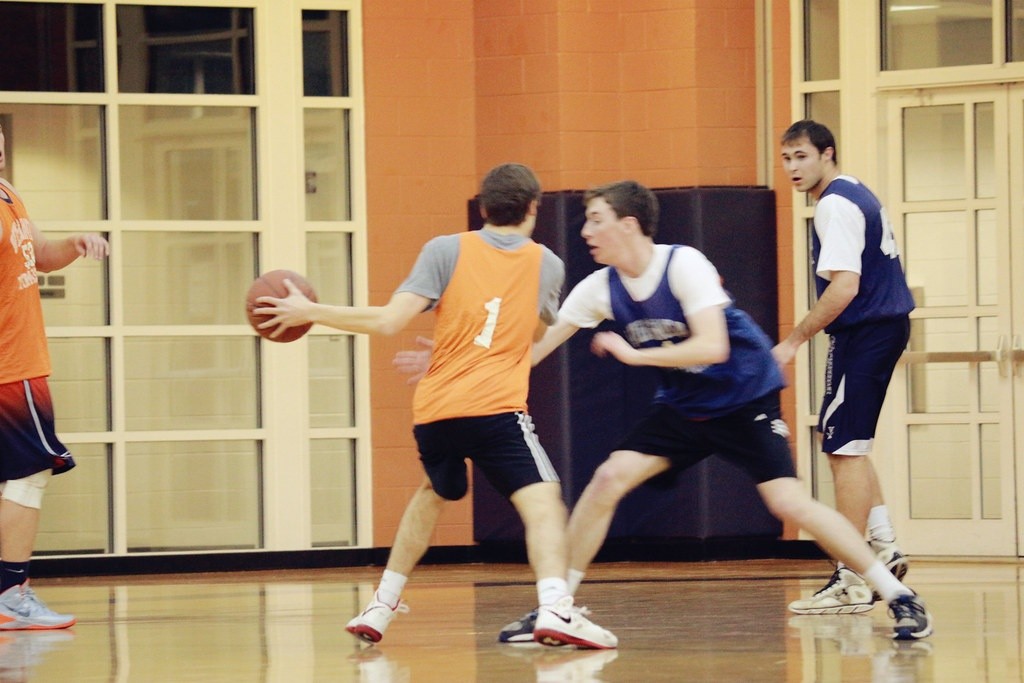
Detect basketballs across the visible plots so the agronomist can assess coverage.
[245,269,317,343]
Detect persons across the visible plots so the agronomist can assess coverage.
[0,129,113,630]
[770,117,916,615]
[253,161,619,647]
[0,631,78,683]
[392,181,932,643]
[786,613,933,683]
[346,642,617,683]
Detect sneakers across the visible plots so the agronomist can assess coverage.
[788,561,875,615]
[347,648,386,671]
[534,595,618,649]
[346,590,402,642]
[0,578,76,630]
[867,537,908,601]
[0,629,76,668]
[887,586,933,640]
[534,650,618,683]
[499,608,538,641]
